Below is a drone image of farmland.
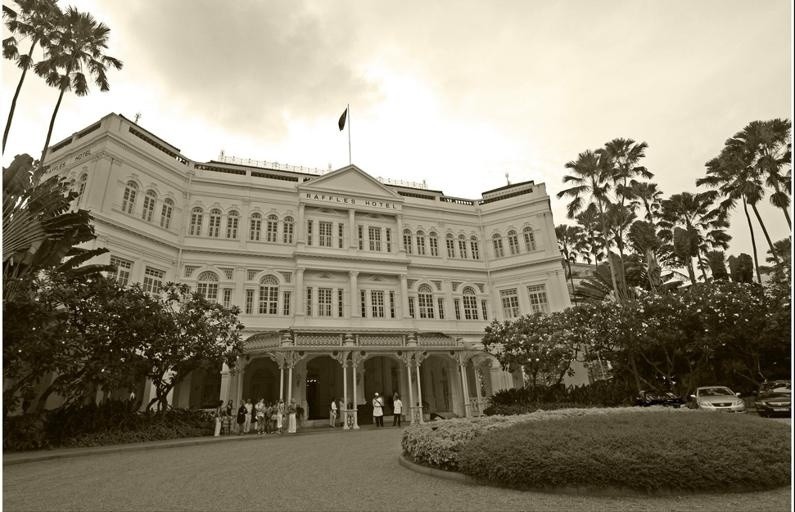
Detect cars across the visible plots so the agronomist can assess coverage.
[690,385,745,413]
[752,379,791,417]
[632,389,686,408]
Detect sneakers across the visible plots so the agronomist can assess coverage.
[392,424,401,426]
[214,431,282,437]
[376,425,384,427]
[329,425,336,428]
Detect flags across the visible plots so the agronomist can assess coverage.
[337,106,348,131]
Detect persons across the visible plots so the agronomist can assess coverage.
[392,392,403,427]
[338,396,345,426]
[213,397,298,438]
[328,397,338,428]
[371,392,385,427]
